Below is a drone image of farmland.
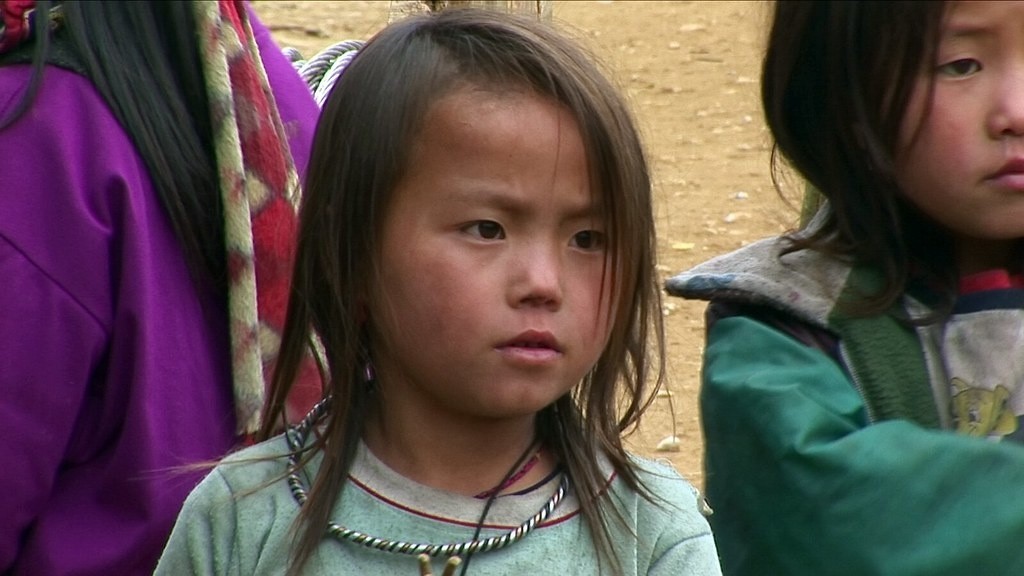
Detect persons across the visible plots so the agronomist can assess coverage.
[665,0,1024,576]
[153,8,724,576]
[0,0,333,576]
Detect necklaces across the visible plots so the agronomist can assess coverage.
[286,393,572,557]
